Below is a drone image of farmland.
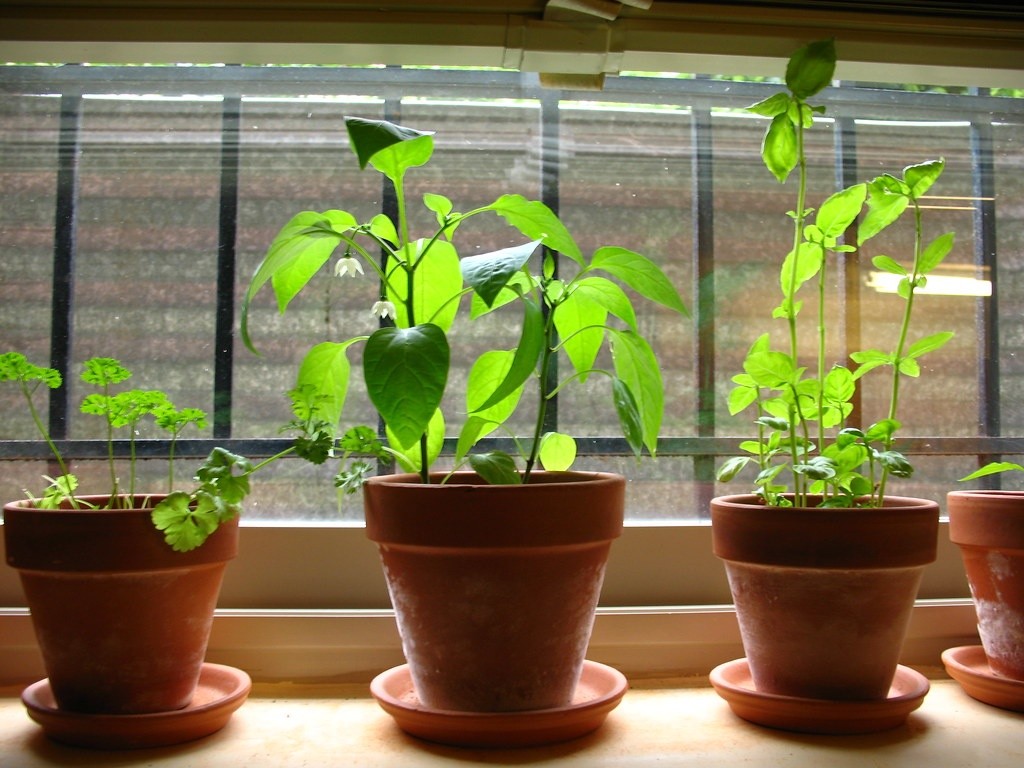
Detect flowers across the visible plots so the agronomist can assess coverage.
[238,114,690,483]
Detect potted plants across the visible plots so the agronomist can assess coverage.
[0,347,253,749]
[940,445,1024,711]
[709,35,940,732]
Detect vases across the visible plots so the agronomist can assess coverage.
[363,464,629,749]
